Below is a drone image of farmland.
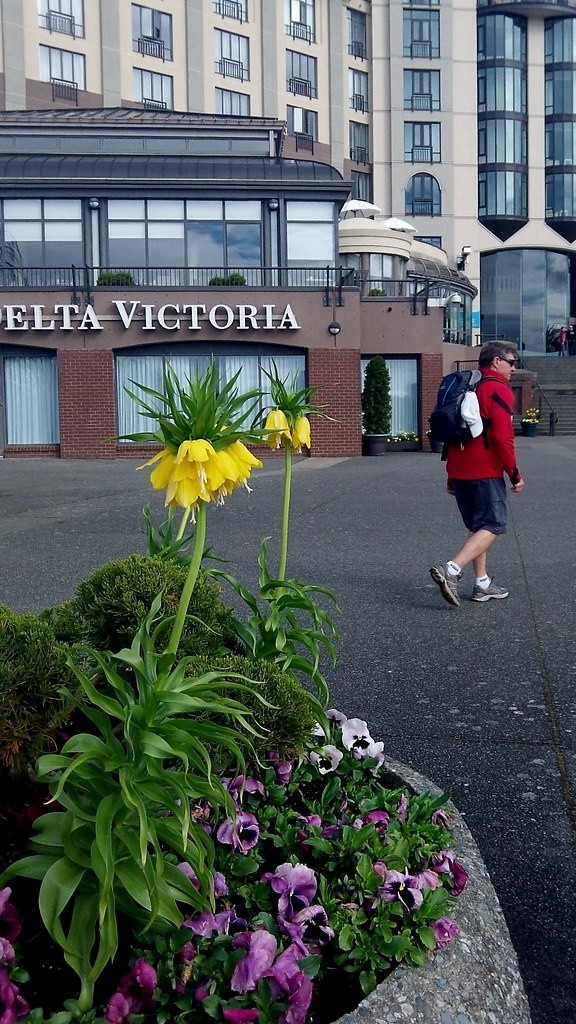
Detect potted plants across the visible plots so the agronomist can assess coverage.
[361,356,392,456]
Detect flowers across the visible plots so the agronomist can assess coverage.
[0,353,469,1024]
[386,430,419,442]
[520,408,540,423]
[425,430,431,439]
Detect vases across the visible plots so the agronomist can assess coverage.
[362,434,390,456]
[298,752,532,1024]
[429,439,444,453]
[520,423,538,437]
[386,442,420,451]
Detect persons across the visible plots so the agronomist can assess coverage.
[556,325,576,357]
[426,341,524,608]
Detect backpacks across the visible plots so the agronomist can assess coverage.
[427,369,510,461]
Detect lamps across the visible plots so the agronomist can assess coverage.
[457,246,471,271]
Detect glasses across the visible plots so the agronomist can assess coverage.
[498,356,516,367]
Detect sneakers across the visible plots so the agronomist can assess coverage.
[429,563,462,607]
[471,575,509,602]
